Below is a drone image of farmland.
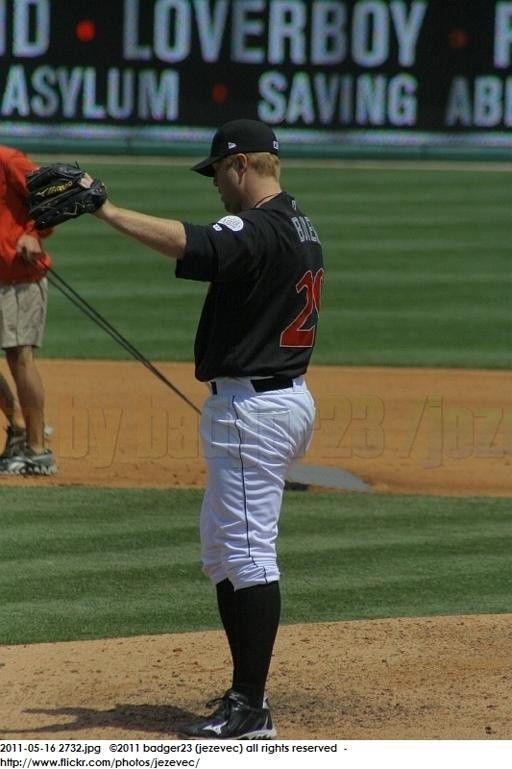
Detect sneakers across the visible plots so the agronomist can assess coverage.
[179,689,277,740]
[0,425,57,475]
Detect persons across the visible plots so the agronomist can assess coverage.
[0,143,65,477]
[31,119,324,740]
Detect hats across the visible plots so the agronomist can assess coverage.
[192,119,279,176]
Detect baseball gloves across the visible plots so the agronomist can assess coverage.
[24,162,107,230]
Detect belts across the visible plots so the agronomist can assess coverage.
[211,378,293,394]
